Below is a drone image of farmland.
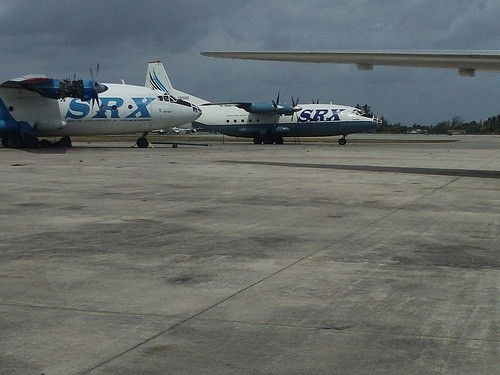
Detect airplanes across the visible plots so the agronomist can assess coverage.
[121,61,382,146]
[0,75,203,147]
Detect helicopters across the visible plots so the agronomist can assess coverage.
[200,49,500,75]
